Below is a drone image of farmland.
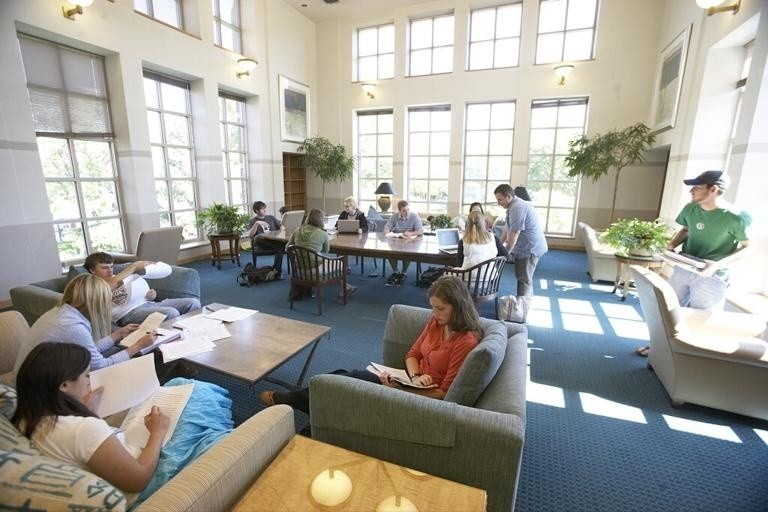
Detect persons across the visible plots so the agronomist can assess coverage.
[10,341,238,512]
[494,184,548,308]
[637,170,749,371]
[11,273,158,380]
[465,202,493,232]
[259,274,483,438]
[335,196,369,275]
[383,201,424,284]
[285,208,359,299]
[248,201,287,279]
[83,252,202,378]
[279,205,288,221]
[443,211,509,288]
[514,186,533,201]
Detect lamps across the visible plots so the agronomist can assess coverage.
[360,81,378,100]
[62,0,92,21]
[375,183,394,211]
[554,63,574,86]
[694,0,742,16]
[232,56,259,82]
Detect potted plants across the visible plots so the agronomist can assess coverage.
[602,214,673,254]
[197,204,249,230]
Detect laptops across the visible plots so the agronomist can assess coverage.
[338,220,360,233]
[436,229,460,255]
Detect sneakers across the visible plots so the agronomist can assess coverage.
[168,360,200,379]
[383,272,408,289]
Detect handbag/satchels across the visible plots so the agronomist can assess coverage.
[497,294,532,323]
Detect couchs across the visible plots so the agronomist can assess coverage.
[308,303,529,512]
[629,265,768,427]
[579,220,656,284]
[8,258,200,343]
[103,224,185,264]
[0,311,296,512]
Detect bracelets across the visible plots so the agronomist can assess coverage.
[410,373,422,380]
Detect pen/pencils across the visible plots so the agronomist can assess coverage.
[146,332,167,337]
[173,326,183,330]
[206,307,215,312]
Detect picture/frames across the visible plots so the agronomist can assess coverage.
[278,72,312,145]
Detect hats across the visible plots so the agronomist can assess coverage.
[682,169,732,192]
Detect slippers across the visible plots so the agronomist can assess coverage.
[339,285,358,298]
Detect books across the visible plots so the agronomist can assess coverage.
[137,327,182,355]
[365,361,439,390]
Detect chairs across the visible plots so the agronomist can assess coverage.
[285,244,348,316]
[438,256,509,316]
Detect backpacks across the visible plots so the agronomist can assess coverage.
[417,266,445,289]
[235,262,280,288]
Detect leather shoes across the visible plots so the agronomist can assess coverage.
[261,390,297,407]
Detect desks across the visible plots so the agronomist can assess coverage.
[612,252,662,304]
[133,301,331,410]
[205,230,243,272]
[246,203,516,291]
[230,435,488,512]
